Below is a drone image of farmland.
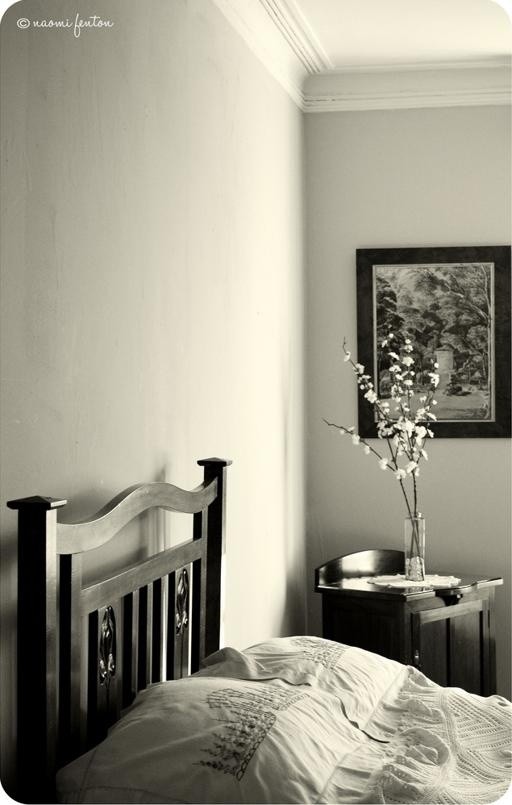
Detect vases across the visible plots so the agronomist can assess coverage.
[404,516,428,583]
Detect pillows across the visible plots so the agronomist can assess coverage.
[197,635,425,742]
[79,675,385,804]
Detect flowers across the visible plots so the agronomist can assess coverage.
[323,328,442,578]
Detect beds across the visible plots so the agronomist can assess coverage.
[5,455,510,805]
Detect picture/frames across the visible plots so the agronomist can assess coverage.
[354,244,512,441]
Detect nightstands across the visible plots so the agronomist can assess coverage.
[312,546,506,700]
